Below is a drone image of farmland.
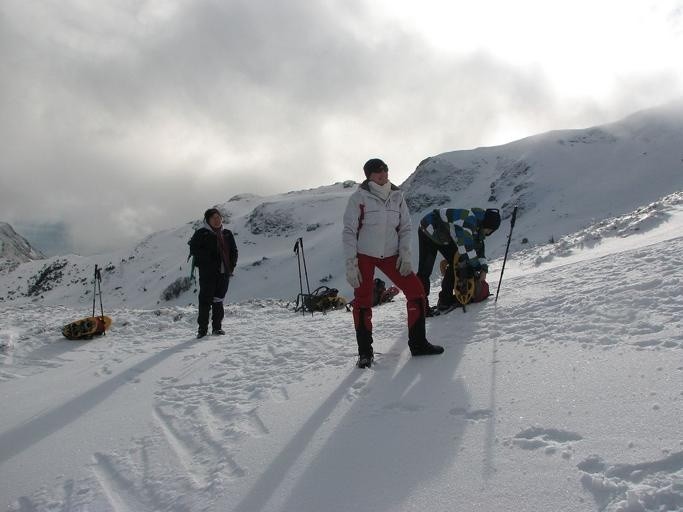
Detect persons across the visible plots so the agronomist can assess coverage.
[186,207,237,340]
[414,207,501,317]
[342,157,444,369]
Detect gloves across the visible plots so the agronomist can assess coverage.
[395,256,413,278]
[345,258,362,289]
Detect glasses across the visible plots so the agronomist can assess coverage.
[373,167,388,173]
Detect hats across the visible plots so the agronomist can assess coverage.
[484,211,500,230]
[363,159,386,177]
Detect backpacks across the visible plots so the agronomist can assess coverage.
[453,253,490,305]
[60,317,104,339]
[344,278,386,312]
[294,286,338,312]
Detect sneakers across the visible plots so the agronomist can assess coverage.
[410,342,444,356]
[358,351,373,369]
[424,295,460,317]
[197,329,225,338]
